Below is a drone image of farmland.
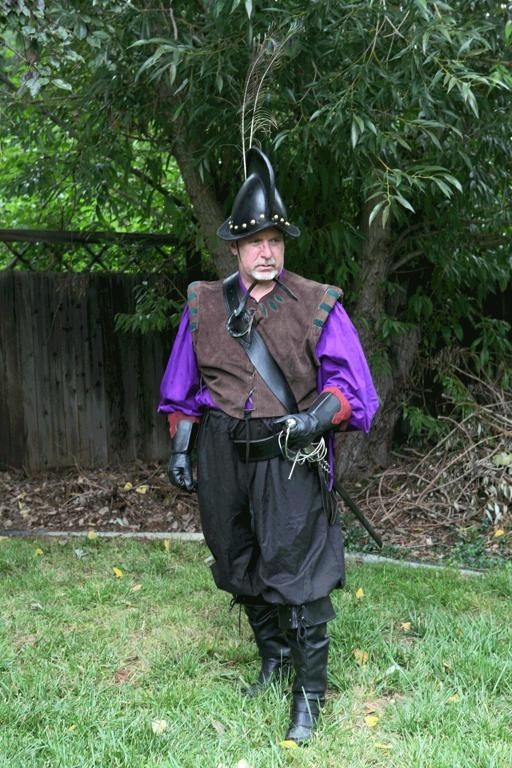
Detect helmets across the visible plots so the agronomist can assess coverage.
[217,146,302,240]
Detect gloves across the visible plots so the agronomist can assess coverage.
[167,419,198,493]
[274,392,342,448]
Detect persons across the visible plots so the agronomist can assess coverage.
[158,146,383,751]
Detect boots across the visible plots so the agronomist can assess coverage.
[229,589,292,698]
[280,597,336,749]
